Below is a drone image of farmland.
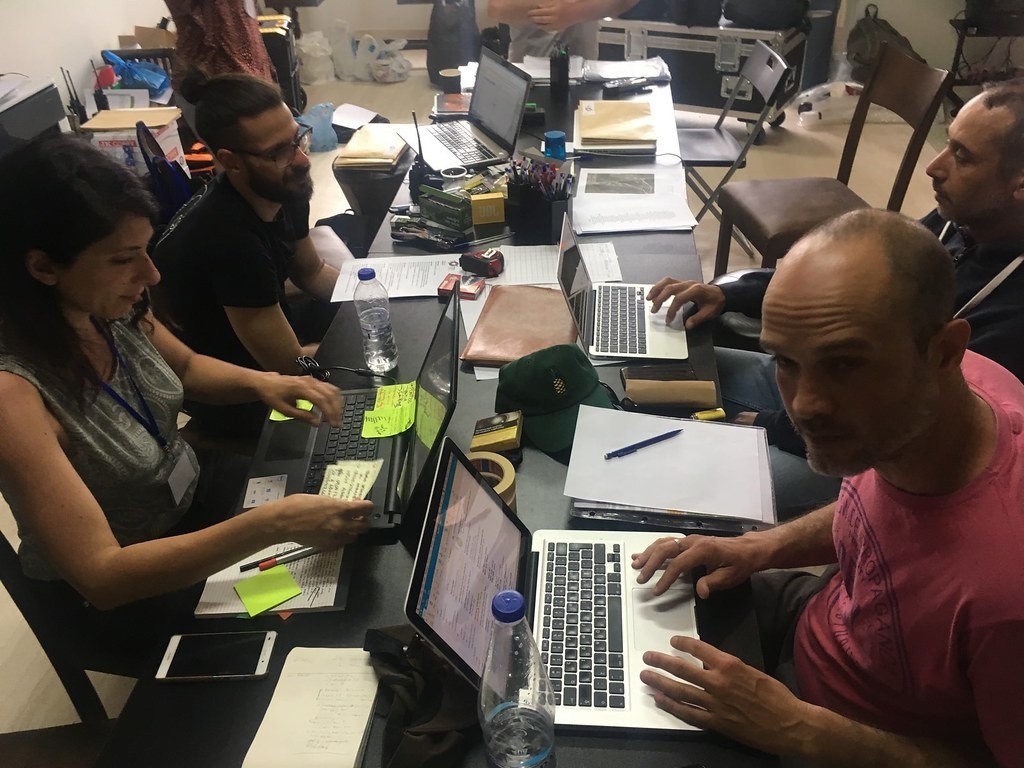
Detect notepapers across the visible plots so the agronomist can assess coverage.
[470,411,522,451]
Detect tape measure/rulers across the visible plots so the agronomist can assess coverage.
[460,249,505,278]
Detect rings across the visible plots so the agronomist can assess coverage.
[674,539,681,545]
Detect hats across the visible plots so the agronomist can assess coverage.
[495,344,617,454]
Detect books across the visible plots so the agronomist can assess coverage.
[572,100,657,153]
[335,123,418,171]
[437,93,472,113]
[460,286,576,368]
[241,647,381,768]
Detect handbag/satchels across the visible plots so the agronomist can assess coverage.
[295,103,338,152]
[296,20,412,85]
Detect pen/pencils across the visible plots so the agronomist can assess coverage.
[506,155,576,201]
[239,546,316,572]
[605,429,685,460]
[456,232,518,248]
[551,42,567,54]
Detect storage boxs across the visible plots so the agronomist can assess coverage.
[89,114,190,186]
[437,274,486,300]
[469,409,523,452]
[470,193,505,225]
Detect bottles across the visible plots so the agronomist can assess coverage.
[354,268,399,373]
[428,352,451,393]
[478,589,555,768]
[545,131,566,162]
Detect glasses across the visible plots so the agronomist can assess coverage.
[218,122,313,168]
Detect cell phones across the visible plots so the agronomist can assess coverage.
[155,631,278,679]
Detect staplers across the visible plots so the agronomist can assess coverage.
[391,231,455,254]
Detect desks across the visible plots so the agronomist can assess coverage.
[88,79,783,768]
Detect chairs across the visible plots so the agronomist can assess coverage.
[712,40,952,280]
[674,39,792,260]
[0,530,162,753]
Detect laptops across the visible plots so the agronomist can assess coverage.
[557,212,689,361]
[405,435,706,731]
[235,281,461,540]
[395,45,533,171]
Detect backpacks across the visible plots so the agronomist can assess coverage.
[847,5,888,84]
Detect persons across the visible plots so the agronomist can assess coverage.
[486,0,641,57]
[649,73,1024,522]
[148,70,341,436]
[0,139,372,611]
[165,0,273,85]
[631,206,1024,768]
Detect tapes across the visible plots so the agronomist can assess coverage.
[463,451,516,517]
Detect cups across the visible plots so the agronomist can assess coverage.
[549,58,570,95]
[439,69,461,94]
[441,166,467,192]
